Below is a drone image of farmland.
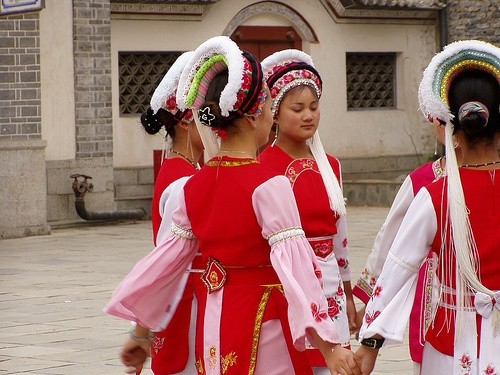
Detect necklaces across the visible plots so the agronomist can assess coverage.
[456,162,499,171]
[169,147,198,171]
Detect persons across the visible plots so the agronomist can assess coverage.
[218,149,256,160]
[352,78,449,343]
[102,37,355,375]
[141,50,199,375]
[353,41,500,375]
[256,48,357,375]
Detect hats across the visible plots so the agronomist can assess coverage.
[174,35,266,140]
[150,50,195,130]
[417,39,500,124]
[260,48,323,119]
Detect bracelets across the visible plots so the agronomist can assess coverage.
[129,331,148,344]
[323,343,340,355]
[362,337,384,349]
